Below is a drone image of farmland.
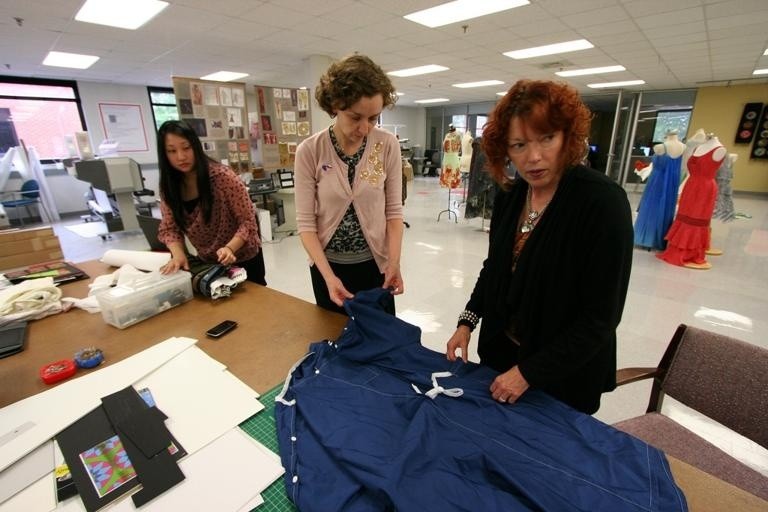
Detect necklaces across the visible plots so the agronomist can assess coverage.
[519,185,557,233]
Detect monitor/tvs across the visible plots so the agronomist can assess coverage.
[639,145,651,157]
[588,145,597,153]
[651,109,692,144]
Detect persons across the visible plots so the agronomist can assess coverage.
[294,55,405,317]
[633,128,738,266]
[439,125,461,188]
[157,120,267,286]
[461,129,475,173]
[447,78,634,416]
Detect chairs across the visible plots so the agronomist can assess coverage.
[607,323,768,502]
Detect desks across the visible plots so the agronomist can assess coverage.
[0,258,768,512]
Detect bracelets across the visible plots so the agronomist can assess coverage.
[458,310,479,328]
[226,246,234,254]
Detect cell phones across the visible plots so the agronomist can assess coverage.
[206,319,237,339]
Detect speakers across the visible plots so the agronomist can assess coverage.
[750,105,768,160]
[734,103,764,144]
